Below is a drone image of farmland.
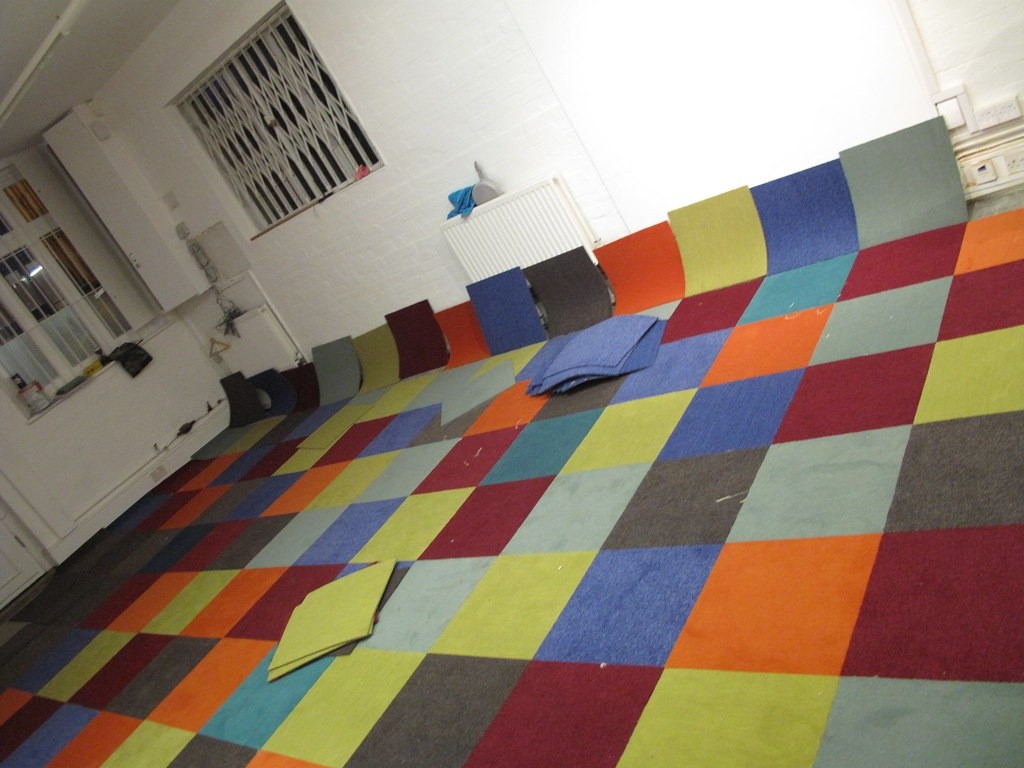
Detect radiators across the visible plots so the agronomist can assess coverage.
[437,178,598,286]
[211,307,299,380]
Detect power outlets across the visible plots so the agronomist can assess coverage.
[1002,149,1023,175]
[973,96,1021,129]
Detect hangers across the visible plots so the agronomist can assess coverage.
[204,335,230,357]
